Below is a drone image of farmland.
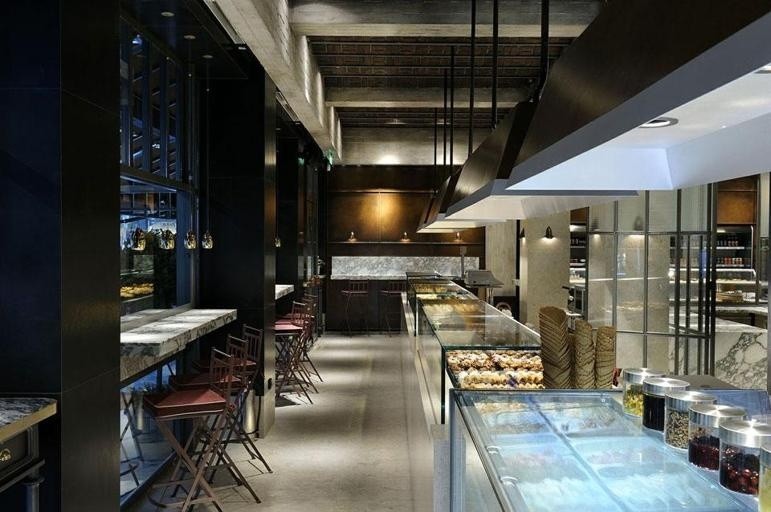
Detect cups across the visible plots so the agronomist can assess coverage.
[717,256,744,264]
[716,240,739,246]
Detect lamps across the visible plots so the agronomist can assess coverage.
[126,10,217,251]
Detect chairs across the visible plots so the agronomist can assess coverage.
[377,277,408,338]
[339,278,371,338]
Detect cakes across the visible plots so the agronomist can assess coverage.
[486,349,543,370]
[507,368,545,390]
[457,368,515,390]
[444,349,494,369]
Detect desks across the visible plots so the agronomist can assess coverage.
[0,395,58,512]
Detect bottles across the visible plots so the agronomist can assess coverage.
[608,368,761,496]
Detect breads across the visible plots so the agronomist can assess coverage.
[505,446,587,482]
[588,444,684,477]
